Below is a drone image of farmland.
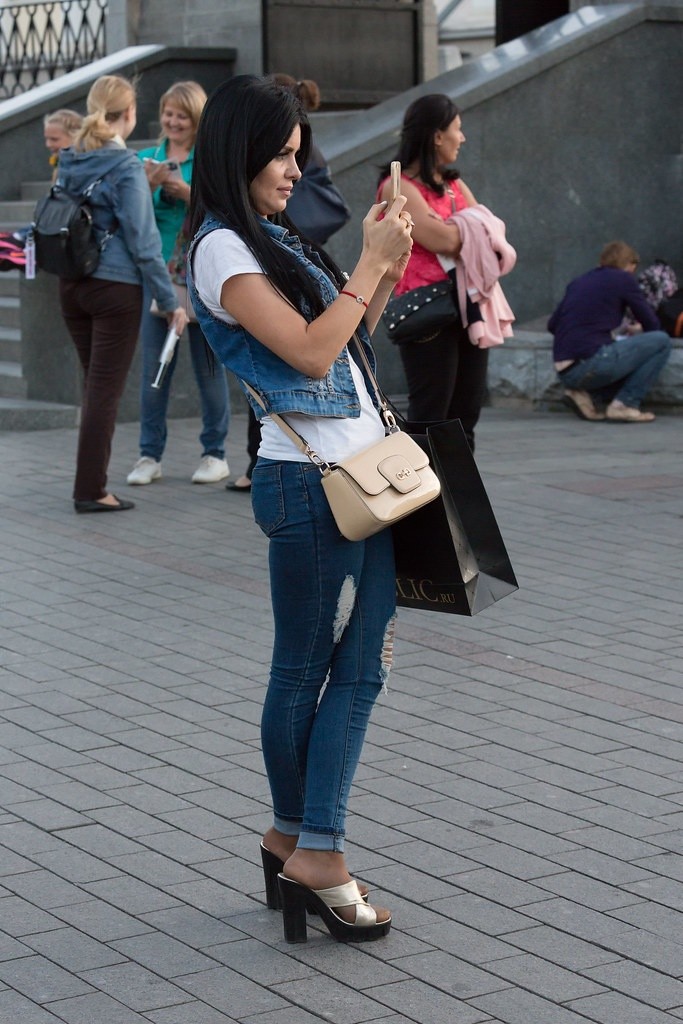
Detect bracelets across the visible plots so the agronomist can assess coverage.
[338,290,368,308]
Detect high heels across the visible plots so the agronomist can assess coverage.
[259,839,367,916]
[276,859,392,943]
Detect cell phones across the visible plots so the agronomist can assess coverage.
[142,157,160,171]
[388,161,401,215]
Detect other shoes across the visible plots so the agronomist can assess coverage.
[605,404,655,422]
[76,492,138,513]
[562,387,606,419]
[225,474,254,491]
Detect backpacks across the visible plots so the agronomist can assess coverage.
[30,152,139,278]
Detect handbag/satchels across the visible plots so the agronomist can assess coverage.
[322,431,441,542]
[390,413,519,617]
[381,278,462,345]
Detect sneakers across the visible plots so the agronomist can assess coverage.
[190,455,230,483]
[127,455,163,486]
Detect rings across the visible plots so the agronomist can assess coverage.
[402,216,408,227]
[410,222,415,229]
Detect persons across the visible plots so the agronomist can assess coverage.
[184,74,413,946]
[376,92,488,456]
[127,81,230,483]
[227,73,352,492]
[546,240,671,422]
[52,75,190,514]
[44,109,86,181]
